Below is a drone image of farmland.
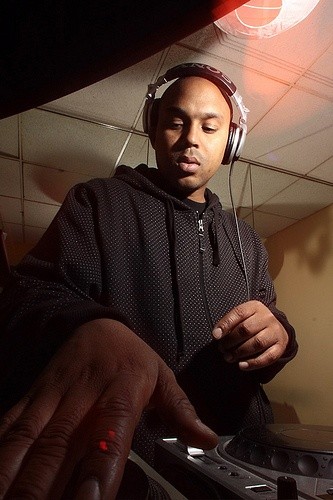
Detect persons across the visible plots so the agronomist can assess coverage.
[0,60,300,500]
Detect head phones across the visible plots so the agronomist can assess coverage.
[143,63,248,165]
